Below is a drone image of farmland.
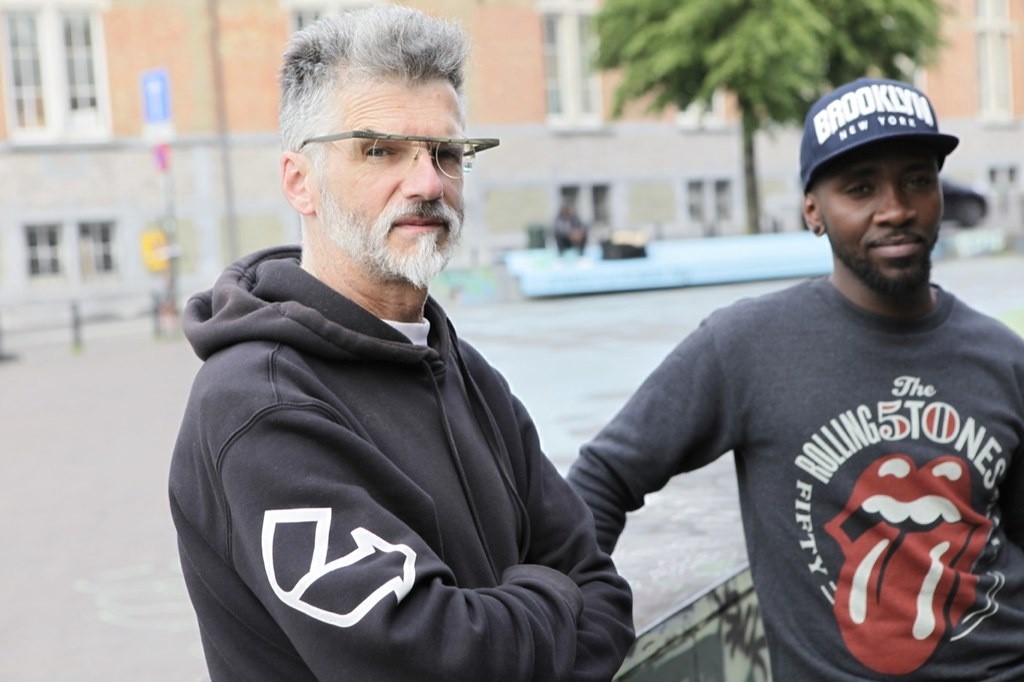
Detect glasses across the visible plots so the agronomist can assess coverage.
[298,132,499,179]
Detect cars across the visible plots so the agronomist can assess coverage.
[941,181,987,227]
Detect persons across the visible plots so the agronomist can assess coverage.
[169,7,635,682]
[567,79,1023,682]
[554,201,587,256]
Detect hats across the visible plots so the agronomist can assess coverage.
[800,78,959,197]
